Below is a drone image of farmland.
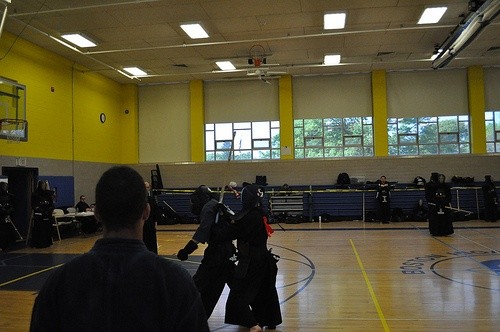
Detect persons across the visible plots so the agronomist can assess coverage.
[75,195,96,238]
[429,175,454,236]
[208,184,282,331]
[0,175,15,252]
[144,181,160,254]
[482,174,498,222]
[31,180,52,247]
[176,184,238,320]
[376,176,391,224]
[30,166,211,332]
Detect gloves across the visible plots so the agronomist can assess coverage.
[216,203,232,223]
[177,240,198,261]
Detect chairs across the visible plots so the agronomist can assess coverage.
[53,209,70,240]
[67,207,81,235]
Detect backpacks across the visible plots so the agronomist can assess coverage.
[337,172,350,189]
[430,172,439,183]
[157,210,200,226]
[242,182,249,188]
[268,212,329,224]
[365,205,473,223]
[30,235,53,248]
[413,176,426,189]
[451,176,475,188]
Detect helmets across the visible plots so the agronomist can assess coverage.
[241,185,265,209]
[190,185,211,207]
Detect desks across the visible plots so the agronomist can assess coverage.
[35,212,94,240]
